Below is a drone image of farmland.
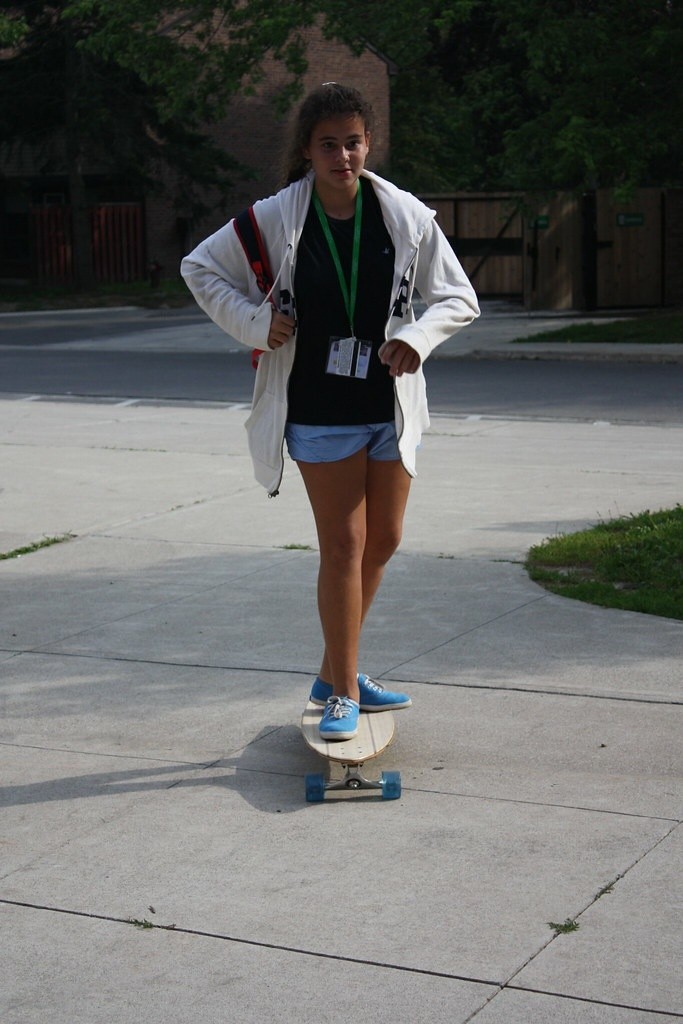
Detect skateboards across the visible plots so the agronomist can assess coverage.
[300,677,402,806]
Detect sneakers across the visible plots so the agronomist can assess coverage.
[308,672,412,712]
[318,696,360,739]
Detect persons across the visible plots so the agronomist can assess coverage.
[180,84,481,739]
